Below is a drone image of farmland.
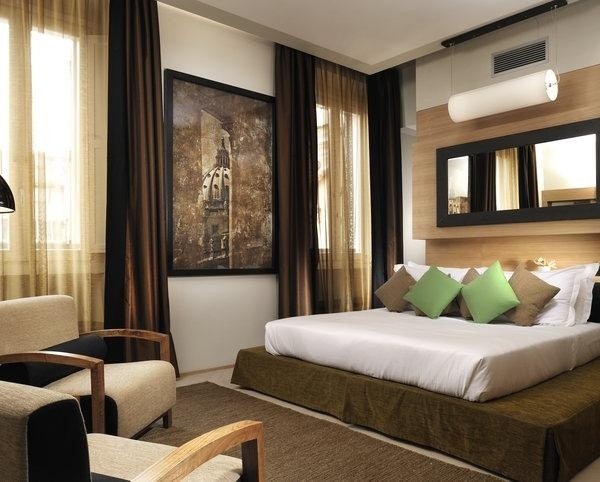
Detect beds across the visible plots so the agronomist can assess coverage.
[231,260,600,481]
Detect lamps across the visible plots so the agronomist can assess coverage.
[441,0,572,122]
[1,173,17,219]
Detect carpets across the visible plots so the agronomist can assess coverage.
[125,380,508,480]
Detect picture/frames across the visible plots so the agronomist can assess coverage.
[163,66,281,276]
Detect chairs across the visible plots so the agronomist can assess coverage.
[2,379,264,482]
[1,294,178,440]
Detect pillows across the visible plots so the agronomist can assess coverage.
[377,256,599,331]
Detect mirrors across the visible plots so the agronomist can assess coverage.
[436,117,599,227]
